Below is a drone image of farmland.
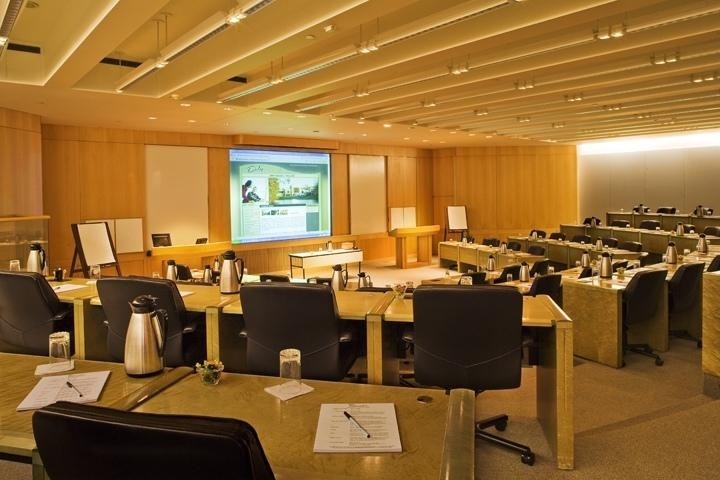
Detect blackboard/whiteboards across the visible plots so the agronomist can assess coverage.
[71,222,118,270]
[447,205,468,229]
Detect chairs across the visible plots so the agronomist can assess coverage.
[529,257,551,281]
[491,265,522,285]
[459,272,488,287]
[706,254,720,274]
[0,270,72,358]
[526,225,642,253]
[633,204,714,218]
[236,282,352,382]
[403,283,536,467]
[619,268,670,367]
[523,273,564,309]
[666,262,705,350]
[581,215,662,231]
[638,251,664,266]
[94,275,203,368]
[33,399,278,480]
[462,235,547,256]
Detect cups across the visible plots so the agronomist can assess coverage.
[676,209,681,215]
[243,268,247,281]
[489,245,493,250]
[576,261,580,269]
[558,238,562,243]
[656,227,660,232]
[280,348,302,389]
[581,241,585,247]
[599,223,602,227]
[319,248,322,253]
[706,240,710,247]
[470,242,472,245]
[690,230,695,236]
[707,211,711,216]
[90,263,101,284]
[266,279,271,282]
[153,272,159,278]
[509,249,512,254]
[308,279,316,283]
[684,248,690,257]
[626,224,630,231]
[507,274,512,283]
[591,266,600,281]
[548,267,554,274]
[620,208,624,213]
[10,260,21,272]
[49,331,71,371]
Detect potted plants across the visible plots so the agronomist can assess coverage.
[195,360,225,386]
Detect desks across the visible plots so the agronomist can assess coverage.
[505,233,637,270]
[0,346,197,467]
[125,368,478,480]
[79,276,241,374]
[562,270,672,372]
[371,293,578,473]
[200,284,392,386]
[558,222,720,255]
[436,238,545,272]
[605,206,719,231]
[30,271,104,361]
[699,270,720,401]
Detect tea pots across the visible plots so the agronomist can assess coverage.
[676,222,684,236]
[487,255,496,271]
[639,204,644,214]
[166,261,180,283]
[214,258,219,270]
[519,262,530,282]
[600,252,613,278]
[591,216,596,228]
[357,272,371,288]
[27,244,46,277]
[122,295,169,377]
[596,237,603,251]
[203,264,214,283]
[696,205,704,217]
[462,236,467,246]
[326,241,333,252]
[331,265,348,291]
[532,230,537,240]
[698,234,707,255]
[219,251,244,294]
[666,242,678,264]
[500,242,508,254]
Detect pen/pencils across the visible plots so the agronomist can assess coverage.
[54,287,61,291]
[67,381,83,398]
[344,411,371,440]
